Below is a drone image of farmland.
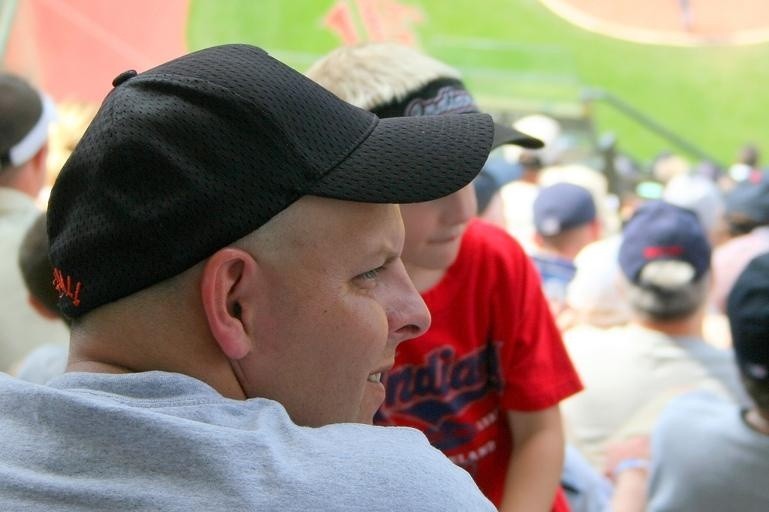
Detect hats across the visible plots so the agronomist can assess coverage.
[503,114,561,168]
[724,253,765,386]
[46,42,495,320]
[618,202,710,296]
[530,181,597,236]
[7,89,85,169]
[722,179,765,224]
[369,76,547,150]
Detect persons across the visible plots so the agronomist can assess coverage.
[295,41,584,512]
[0,40,510,511]
[11,210,68,378]
[1,63,82,382]
